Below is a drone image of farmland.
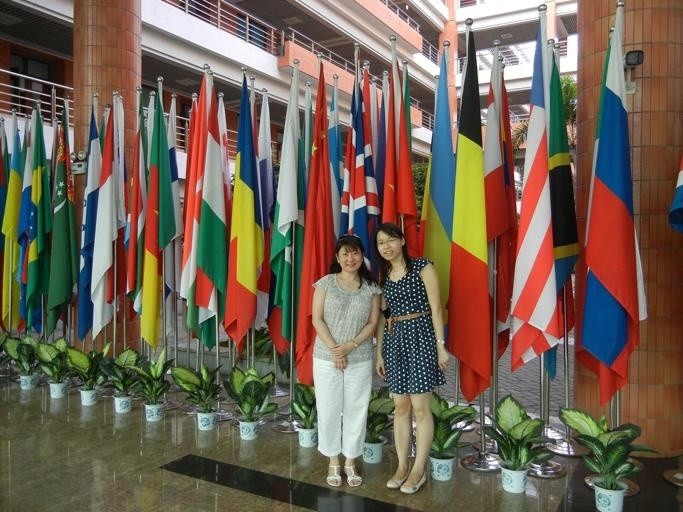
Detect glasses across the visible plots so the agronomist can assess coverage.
[377,238,402,246]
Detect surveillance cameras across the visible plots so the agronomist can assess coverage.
[623,50,644,95]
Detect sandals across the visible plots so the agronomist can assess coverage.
[326,465,342,487]
[344,464,363,487]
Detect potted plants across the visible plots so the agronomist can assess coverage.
[223,365,277,440]
[37,338,70,398]
[560,408,660,512]
[362,390,395,464]
[98,347,137,413]
[67,343,112,407]
[4,336,39,390]
[127,348,175,421]
[483,395,556,494]
[426,393,477,481]
[291,385,317,447]
[171,363,224,431]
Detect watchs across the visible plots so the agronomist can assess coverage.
[436,339,445,345]
[350,339,358,348]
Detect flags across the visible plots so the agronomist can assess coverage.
[224,65,275,360]
[420,40,455,349]
[577,0,647,409]
[668,156,683,234]
[339,35,418,287]
[126,76,184,350]
[0,99,79,341]
[77,89,127,341]
[512,4,579,380]
[447,18,518,403]
[179,64,232,352]
[270,51,342,386]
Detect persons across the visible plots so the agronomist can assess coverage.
[311,235,382,488]
[376,223,451,495]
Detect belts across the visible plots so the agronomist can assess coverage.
[386,310,432,332]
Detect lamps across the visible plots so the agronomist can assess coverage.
[70,151,87,175]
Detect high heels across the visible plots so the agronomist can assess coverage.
[399,472,427,494]
[386,458,413,489]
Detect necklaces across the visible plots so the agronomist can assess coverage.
[391,262,406,273]
